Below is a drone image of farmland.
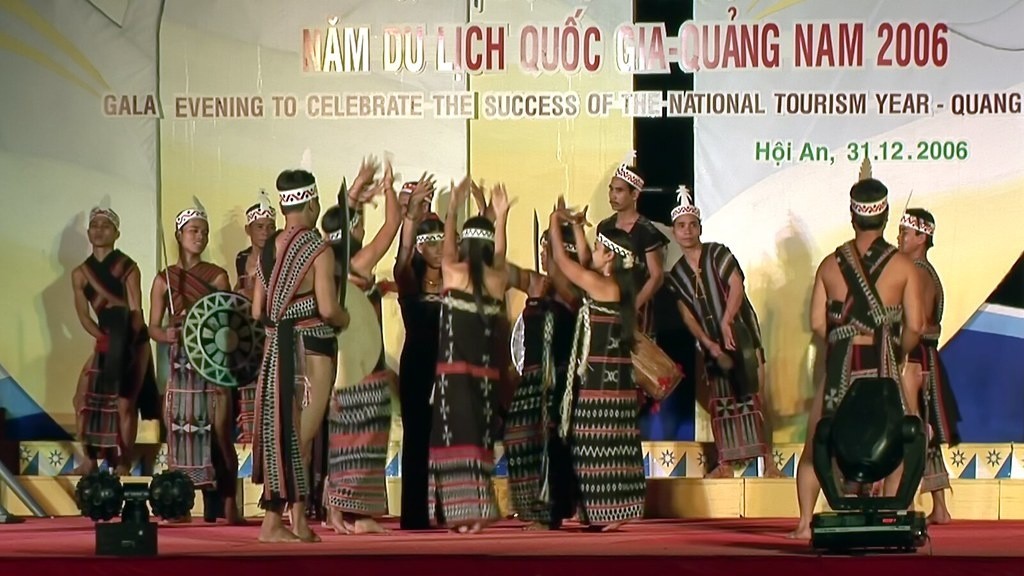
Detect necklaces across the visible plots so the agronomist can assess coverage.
[424,274,440,287]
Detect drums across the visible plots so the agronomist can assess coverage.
[628,327,684,403]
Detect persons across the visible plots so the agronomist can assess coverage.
[786,154,924,541]
[72,147,762,542]
[880,187,959,523]
